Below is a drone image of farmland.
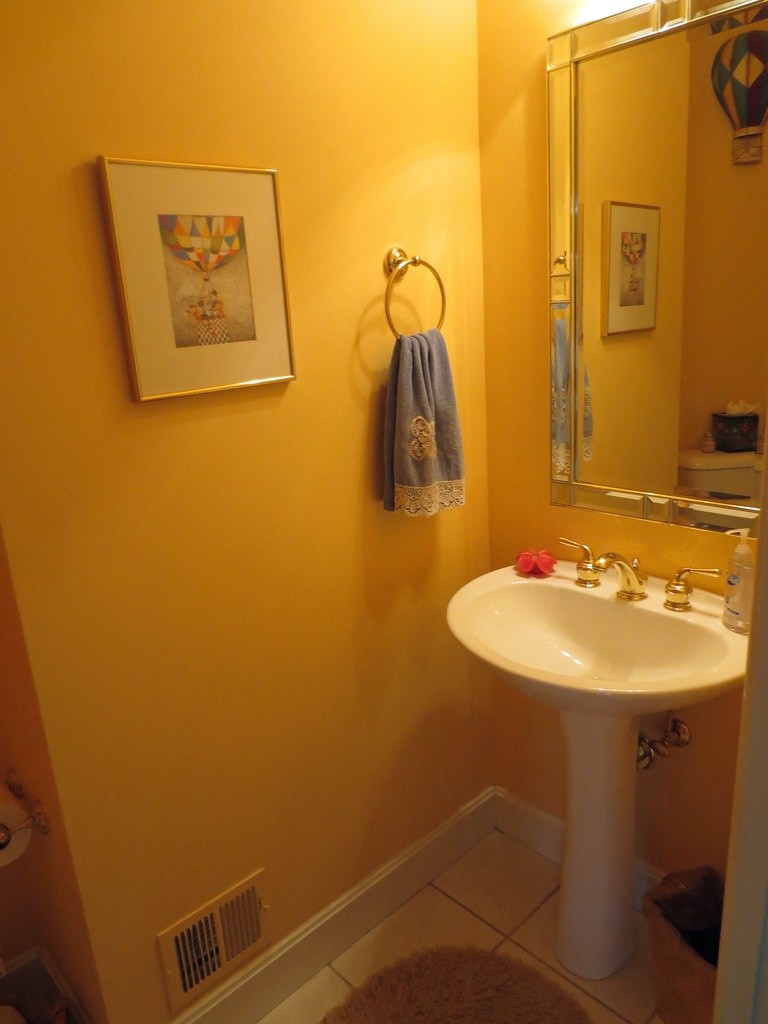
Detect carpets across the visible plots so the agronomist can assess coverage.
[319,946,596,1024]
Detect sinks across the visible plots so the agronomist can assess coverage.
[445,558,750,714]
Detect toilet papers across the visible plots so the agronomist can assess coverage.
[0,781,36,870]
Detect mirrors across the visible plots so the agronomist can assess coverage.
[545,0,766,544]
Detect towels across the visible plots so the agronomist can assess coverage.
[383,328,465,521]
[551,315,593,478]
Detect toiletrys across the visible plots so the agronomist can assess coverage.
[723,526,754,635]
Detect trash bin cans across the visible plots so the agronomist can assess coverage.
[637,862,726,1024]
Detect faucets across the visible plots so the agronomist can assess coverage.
[591,551,649,603]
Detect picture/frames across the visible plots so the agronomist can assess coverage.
[601,200,661,339]
[98,155,297,402]
